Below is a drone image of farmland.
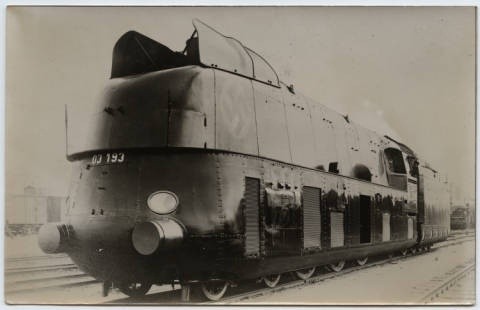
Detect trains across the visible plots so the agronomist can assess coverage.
[38,17,452,303]
